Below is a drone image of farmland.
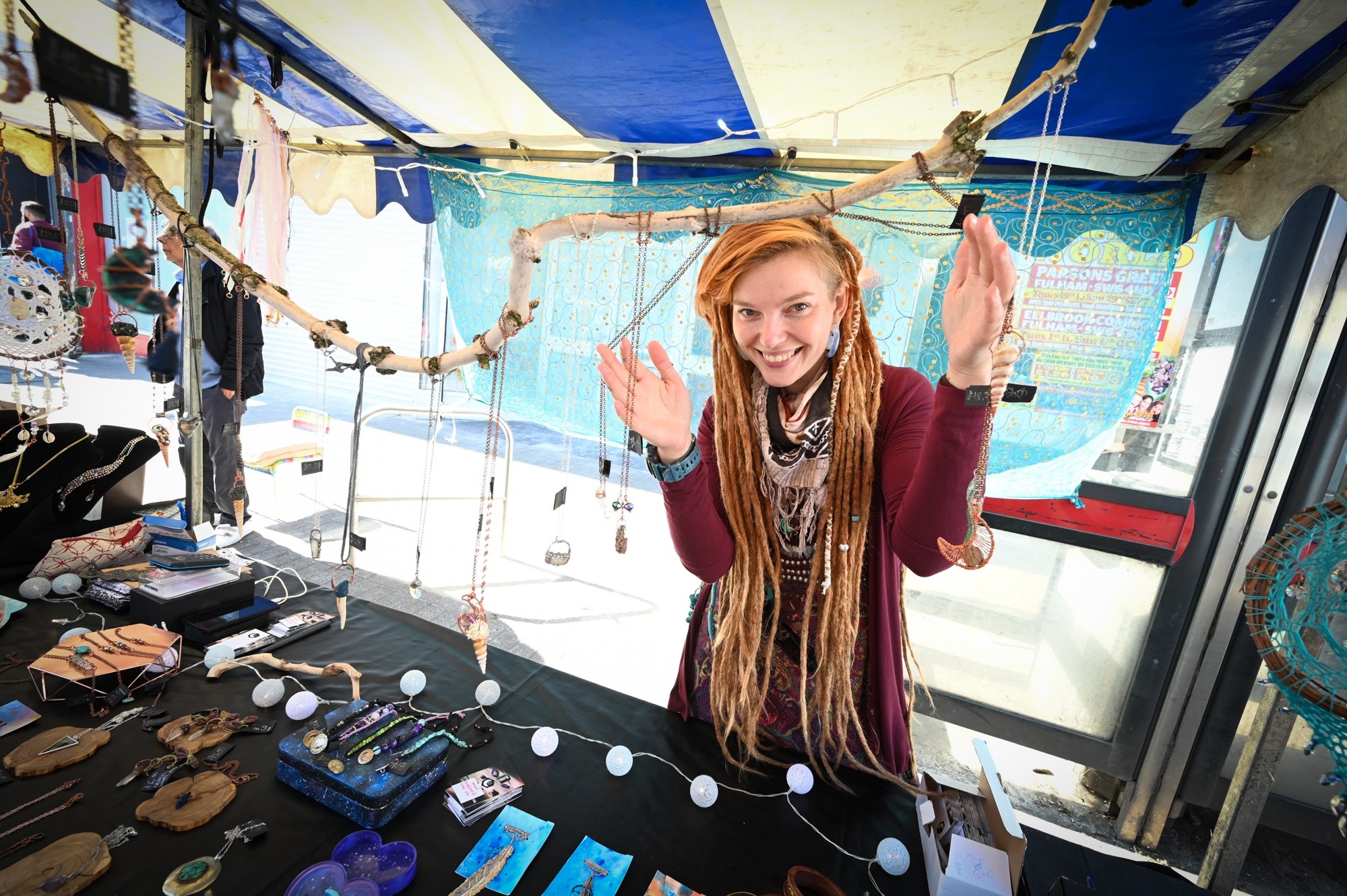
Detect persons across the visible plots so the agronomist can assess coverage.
[1125,355,1175,422]
[8,202,85,366]
[595,212,1014,779]
[155,221,266,550]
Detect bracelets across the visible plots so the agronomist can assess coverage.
[646,432,702,484]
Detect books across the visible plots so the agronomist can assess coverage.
[143,522,194,541]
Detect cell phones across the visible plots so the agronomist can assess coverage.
[183,596,280,633]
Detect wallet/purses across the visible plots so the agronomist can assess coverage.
[24,515,161,581]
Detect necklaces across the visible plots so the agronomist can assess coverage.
[330,341,369,631]
[0,566,608,896]
[814,153,1030,573]
[299,326,330,562]
[405,350,457,600]
[460,205,726,676]
[3,89,256,541]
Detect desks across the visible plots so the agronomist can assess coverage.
[0,528,1212,896]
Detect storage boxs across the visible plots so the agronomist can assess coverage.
[913,736,1027,896]
